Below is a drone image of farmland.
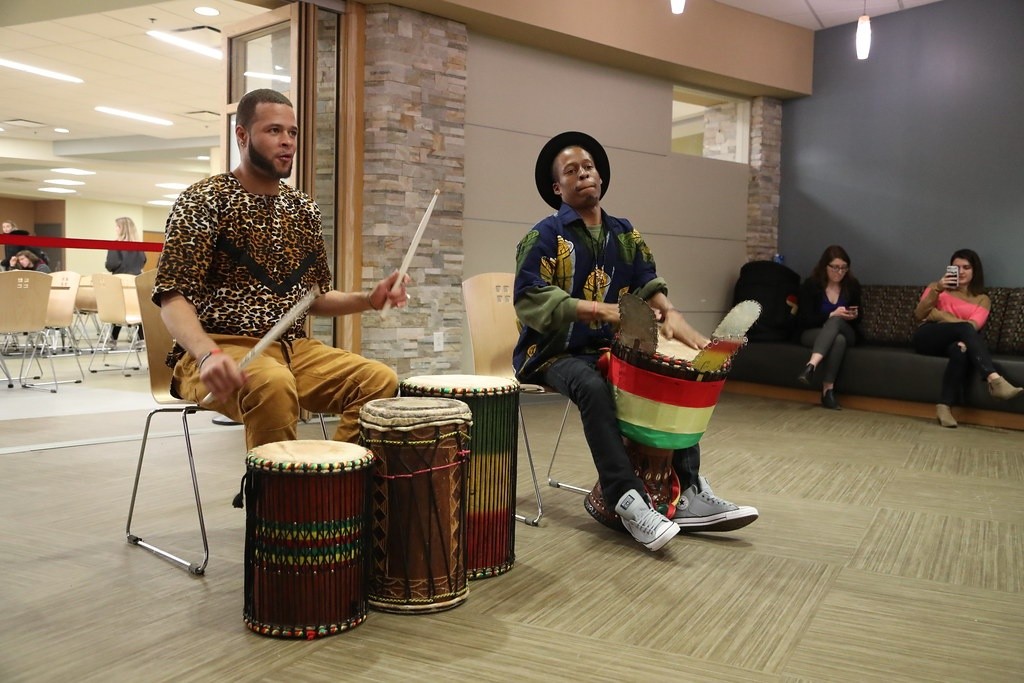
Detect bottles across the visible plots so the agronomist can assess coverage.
[774,254,784,264]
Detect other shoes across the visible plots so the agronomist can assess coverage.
[129,340,146,351]
[104,336,117,350]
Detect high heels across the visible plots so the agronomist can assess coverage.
[936,404,958,427]
[989,376,1023,402]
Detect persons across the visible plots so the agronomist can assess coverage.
[510,131,760,553]
[913,249,1024,427]
[797,246,864,411]
[104,217,147,350]
[151,88,410,448]
[0,220,52,274]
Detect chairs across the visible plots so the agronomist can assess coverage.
[0,270,141,391]
[461,271,591,523]
[126,269,330,574]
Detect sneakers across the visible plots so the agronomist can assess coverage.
[614,489,680,552]
[820,389,843,410]
[671,473,759,533]
[798,363,816,385]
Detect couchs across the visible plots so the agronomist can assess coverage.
[724,283,1023,431]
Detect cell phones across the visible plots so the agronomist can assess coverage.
[947,266,959,287]
[847,306,859,312]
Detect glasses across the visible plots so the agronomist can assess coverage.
[828,263,849,273]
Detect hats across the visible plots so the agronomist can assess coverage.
[534,131,610,210]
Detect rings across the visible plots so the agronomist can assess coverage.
[406,294,411,300]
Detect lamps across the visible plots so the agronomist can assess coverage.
[856,0,871,60]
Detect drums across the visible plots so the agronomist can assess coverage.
[583,330,732,539]
[243,439,376,639]
[398,373,521,582]
[356,396,474,616]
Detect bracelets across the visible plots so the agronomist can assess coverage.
[590,301,598,316]
[368,292,379,311]
[198,349,224,368]
[932,285,942,294]
